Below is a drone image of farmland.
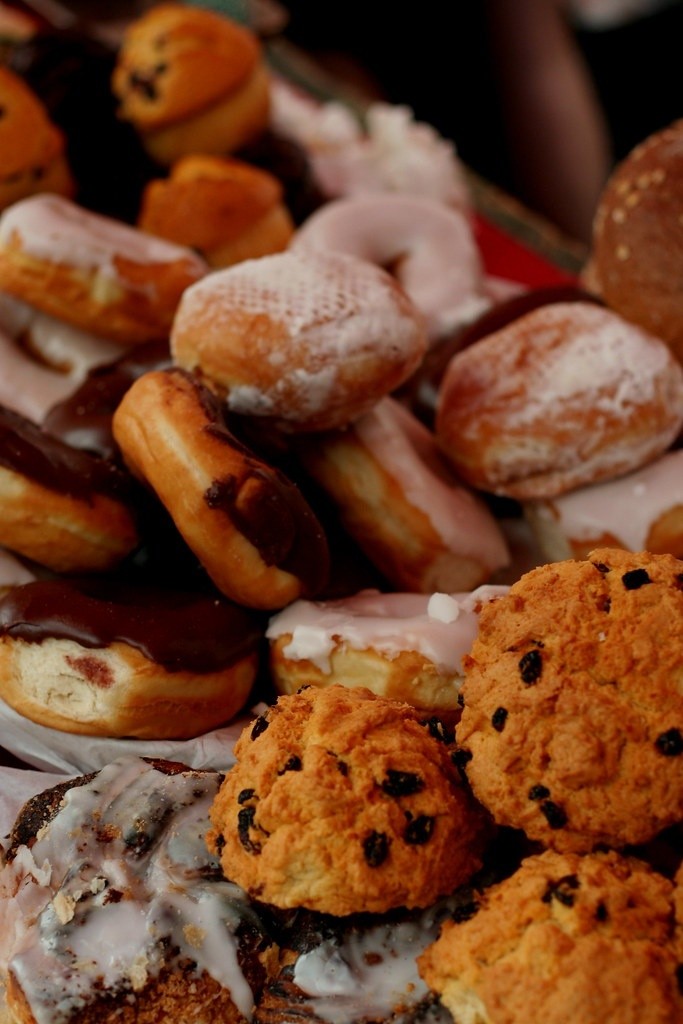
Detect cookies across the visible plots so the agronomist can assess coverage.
[203,549,683,1024]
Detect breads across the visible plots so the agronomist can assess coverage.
[0,0,682,742]
